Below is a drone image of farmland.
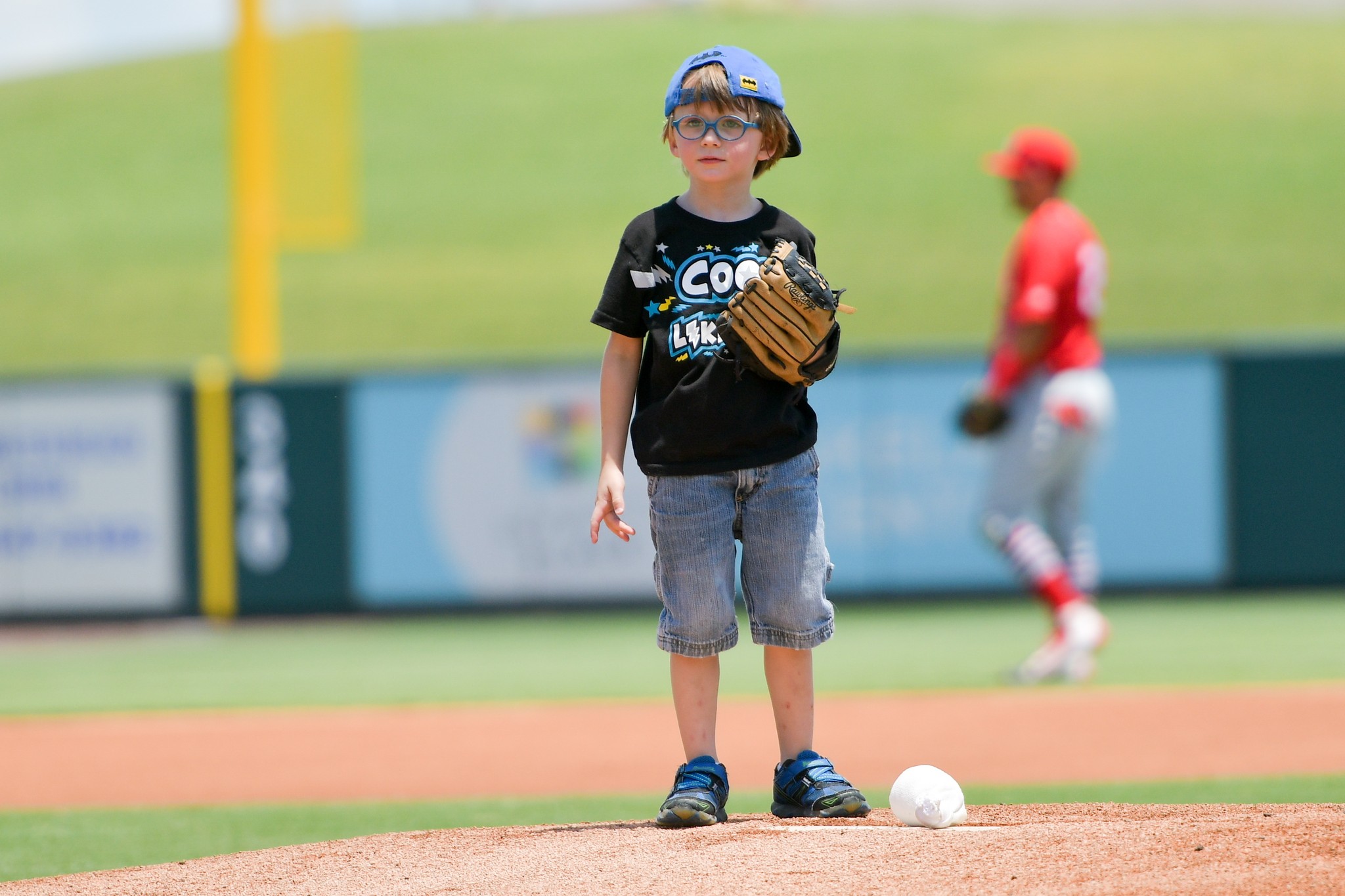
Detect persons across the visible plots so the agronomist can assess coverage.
[961,127,1115,686]
[588,49,874,824]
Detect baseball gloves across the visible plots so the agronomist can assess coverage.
[712,236,840,391]
[954,400,1015,438]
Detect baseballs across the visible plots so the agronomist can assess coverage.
[892,765,964,827]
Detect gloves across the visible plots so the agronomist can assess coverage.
[961,396,1008,436]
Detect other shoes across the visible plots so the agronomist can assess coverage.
[1061,611,1110,682]
[1021,634,1067,683]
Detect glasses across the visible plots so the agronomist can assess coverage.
[673,114,759,140]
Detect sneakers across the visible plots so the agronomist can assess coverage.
[657,756,729,827]
[770,751,871,818]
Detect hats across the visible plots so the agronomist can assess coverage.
[989,126,1072,195]
[665,46,801,159]
